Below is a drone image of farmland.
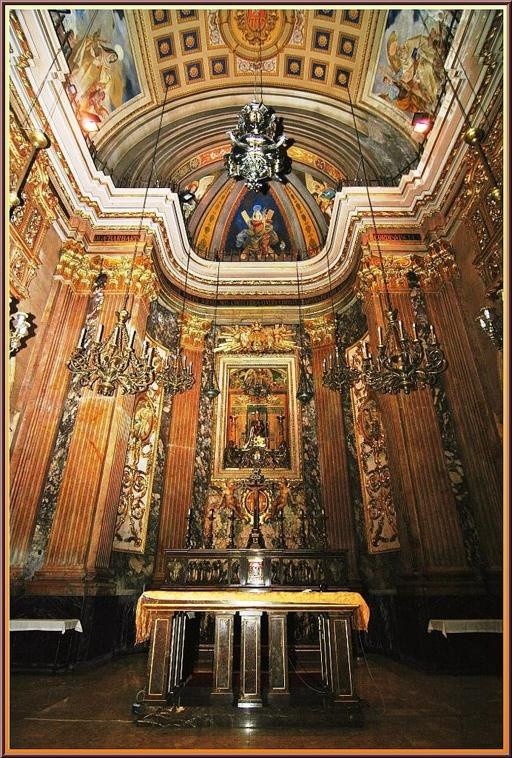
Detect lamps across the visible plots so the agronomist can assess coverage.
[65,67,197,395]
[227,29,291,193]
[294,10,501,406]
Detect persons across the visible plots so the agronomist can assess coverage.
[180,180,201,218]
[71,27,127,115]
[311,190,332,216]
[314,183,338,200]
[382,66,409,100]
[270,477,298,520]
[217,478,246,521]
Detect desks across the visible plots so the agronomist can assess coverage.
[426,615,501,674]
[7,617,83,675]
[129,589,372,731]
